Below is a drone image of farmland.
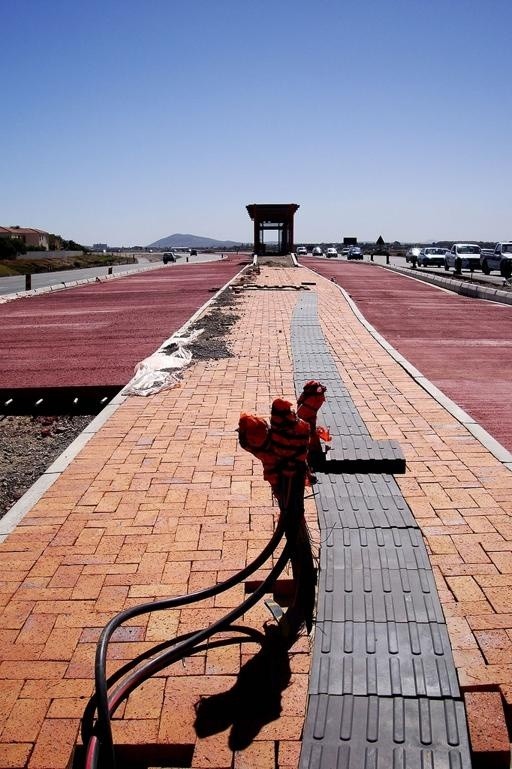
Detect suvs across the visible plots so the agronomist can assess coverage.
[297,247,307,255]
[163,252,176,264]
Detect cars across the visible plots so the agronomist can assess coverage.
[190,250,197,256]
[313,247,323,256]
[406,248,422,264]
[445,243,483,272]
[348,247,364,260]
[418,247,446,268]
[325,248,337,258]
[342,247,349,255]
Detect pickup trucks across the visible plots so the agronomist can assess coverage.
[481,241,512,277]
